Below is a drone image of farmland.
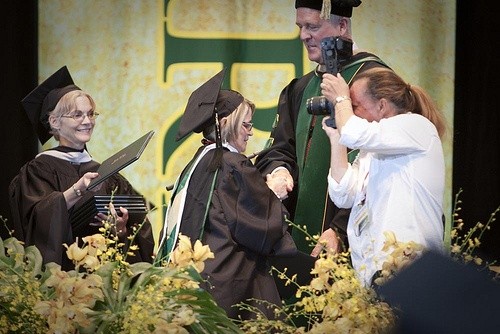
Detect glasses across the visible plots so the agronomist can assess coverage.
[241,121,254,131]
[52,110,100,120]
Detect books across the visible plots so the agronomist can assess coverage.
[71,129,156,237]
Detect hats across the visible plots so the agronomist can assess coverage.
[295,0,361,18]
[19,66,84,146]
[175,67,244,140]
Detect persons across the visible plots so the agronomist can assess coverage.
[158,1,500,334]
[10,65,155,274]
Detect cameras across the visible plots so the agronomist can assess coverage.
[306,35,354,129]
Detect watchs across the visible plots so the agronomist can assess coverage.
[332,96,353,107]
[73,183,83,197]
